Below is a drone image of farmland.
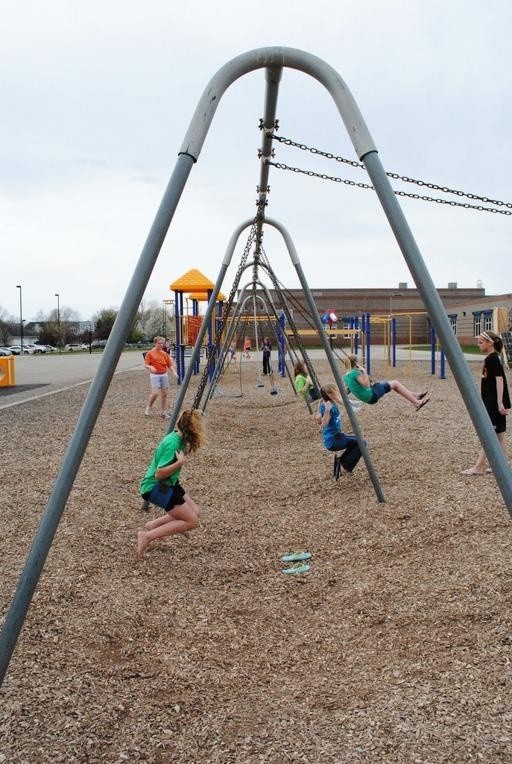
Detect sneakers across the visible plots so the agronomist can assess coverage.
[145,407,151,416]
[160,412,170,419]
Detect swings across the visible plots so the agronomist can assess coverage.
[211,285,255,399]
[257,237,377,454]
[146,220,255,509]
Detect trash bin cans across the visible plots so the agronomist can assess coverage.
[0,355,16,387]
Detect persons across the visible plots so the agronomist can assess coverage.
[244,335,250,359]
[318,382,367,482]
[144,335,180,418]
[165,338,170,353]
[342,353,431,410]
[261,335,273,376]
[459,329,511,475]
[136,407,204,560]
[293,359,363,412]
[230,336,234,359]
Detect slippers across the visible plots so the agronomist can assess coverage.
[416,397,430,411]
[281,550,312,562]
[460,469,484,476]
[418,391,428,400]
[336,460,342,481]
[281,561,310,574]
[334,454,339,476]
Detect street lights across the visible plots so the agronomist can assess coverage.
[388,292,406,358]
[54,293,62,354]
[14,285,25,356]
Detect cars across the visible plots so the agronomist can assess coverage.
[0,337,159,355]
